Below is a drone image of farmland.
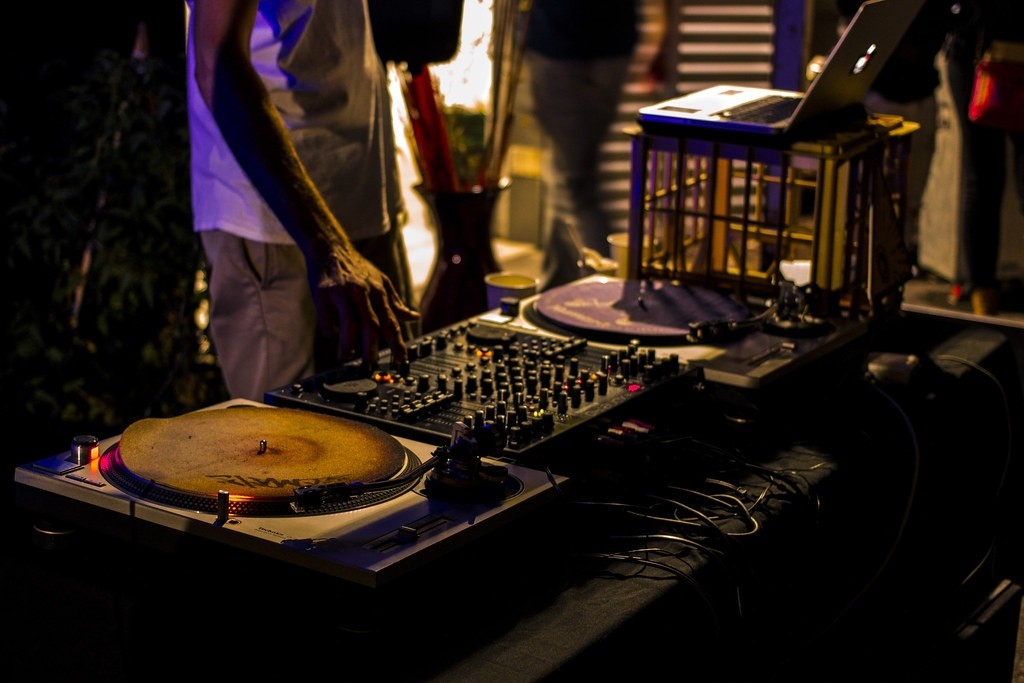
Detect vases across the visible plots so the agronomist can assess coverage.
[412,174,502,336]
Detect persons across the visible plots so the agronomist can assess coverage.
[181,1,423,398]
[837,0,1024,316]
[517,0,639,273]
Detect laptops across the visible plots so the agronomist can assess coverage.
[637,0,926,138]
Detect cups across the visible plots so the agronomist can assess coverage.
[484,273,536,310]
[607,232,628,277]
